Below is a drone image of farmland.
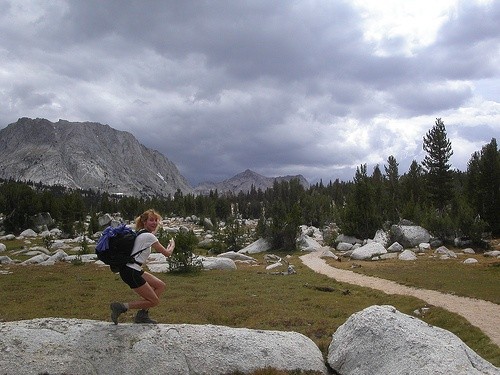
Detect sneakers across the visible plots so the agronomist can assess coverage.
[110,302,127,325]
[135,310,158,324]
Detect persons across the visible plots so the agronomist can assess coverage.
[110,209,175,325]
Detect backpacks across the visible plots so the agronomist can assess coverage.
[94,224,151,274]
